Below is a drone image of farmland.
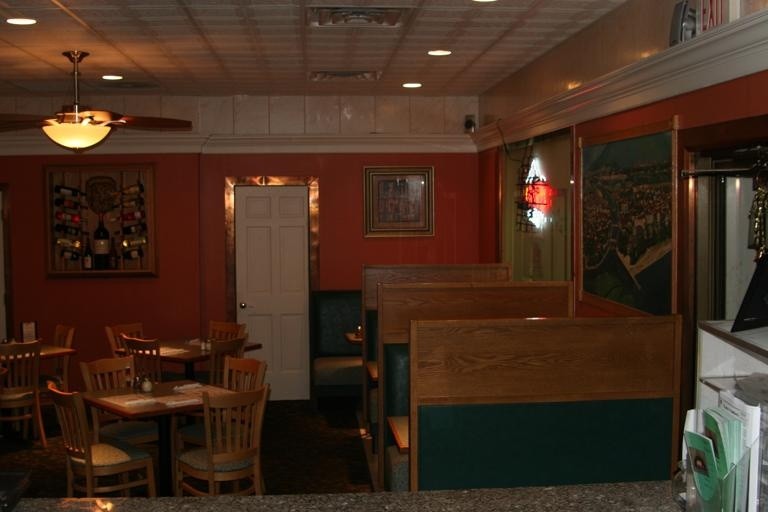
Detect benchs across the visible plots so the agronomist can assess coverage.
[312,263,682,492]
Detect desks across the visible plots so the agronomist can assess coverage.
[114,339,263,372]
[2,345,75,426]
[80,379,239,498]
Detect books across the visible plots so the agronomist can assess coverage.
[683,390,761,512]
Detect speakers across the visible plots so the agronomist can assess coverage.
[669,1,697,46]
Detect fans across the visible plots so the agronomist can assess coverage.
[0,52,193,135]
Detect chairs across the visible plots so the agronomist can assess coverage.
[119,334,161,380]
[0,339,48,450]
[187,356,269,449]
[104,322,145,357]
[171,383,270,498]
[44,324,75,393]
[208,332,250,386]
[47,380,157,497]
[208,319,247,340]
[78,355,161,448]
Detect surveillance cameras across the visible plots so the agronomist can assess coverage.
[463,114,475,134]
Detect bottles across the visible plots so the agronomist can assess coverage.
[93,213,112,271]
[135,375,152,392]
[54,183,85,261]
[200,340,211,351]
[109,183,147,260]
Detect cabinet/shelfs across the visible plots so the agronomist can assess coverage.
[40,159,160,277]
[680,168,768,512]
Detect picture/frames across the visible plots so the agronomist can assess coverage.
[363,165,435,238]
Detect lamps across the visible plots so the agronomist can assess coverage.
[522,157,555,231]
[40,110,110,150]
